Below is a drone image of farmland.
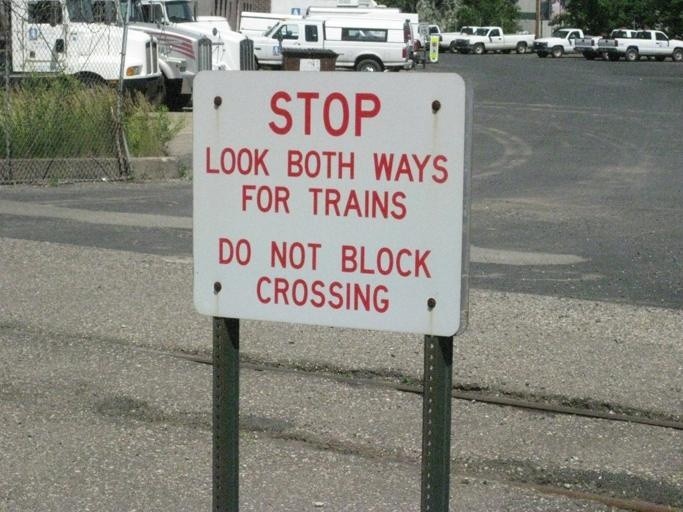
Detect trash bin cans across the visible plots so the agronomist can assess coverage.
[282,49,336,70]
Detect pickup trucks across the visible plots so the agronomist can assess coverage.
[424,23,683,62]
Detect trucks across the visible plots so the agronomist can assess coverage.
[0,0,424,112]
